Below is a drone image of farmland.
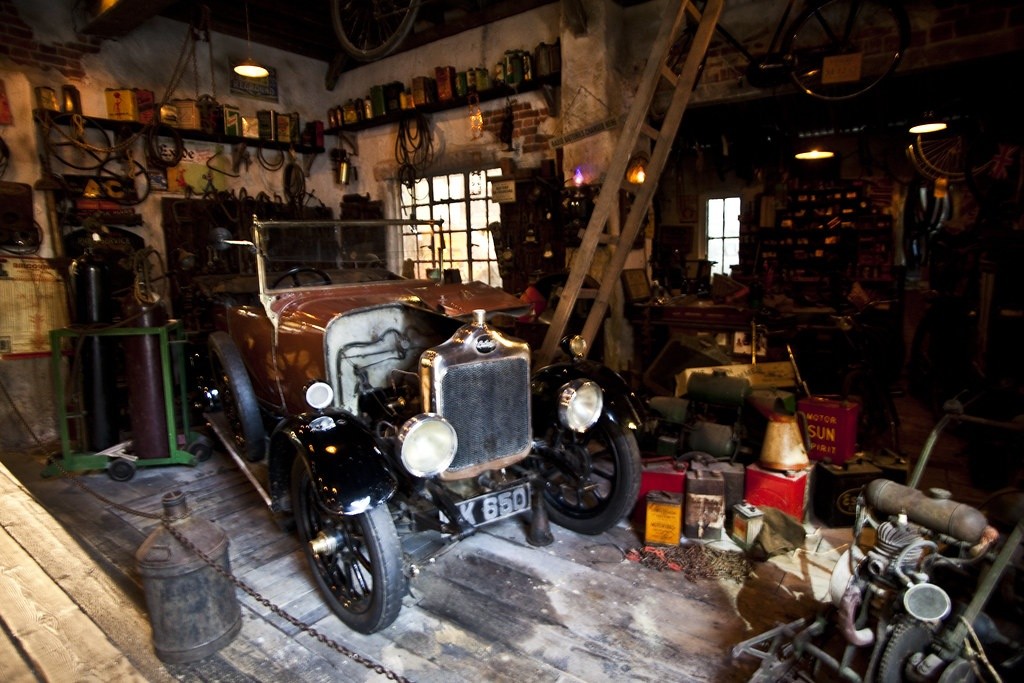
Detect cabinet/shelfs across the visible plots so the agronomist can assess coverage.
[752,188,897,292]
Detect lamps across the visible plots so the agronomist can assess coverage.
[908,103,947,135]
[574,166,584,184]
[233,2,269,77]
[795,144,834,160]
[636,165,646,184]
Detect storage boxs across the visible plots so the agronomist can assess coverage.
[635,359,910,550]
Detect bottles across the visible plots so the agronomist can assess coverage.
[328,35,562,128]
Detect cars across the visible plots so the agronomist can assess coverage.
[163,211,641,634]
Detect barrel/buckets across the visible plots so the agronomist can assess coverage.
[138,485,244,666]
